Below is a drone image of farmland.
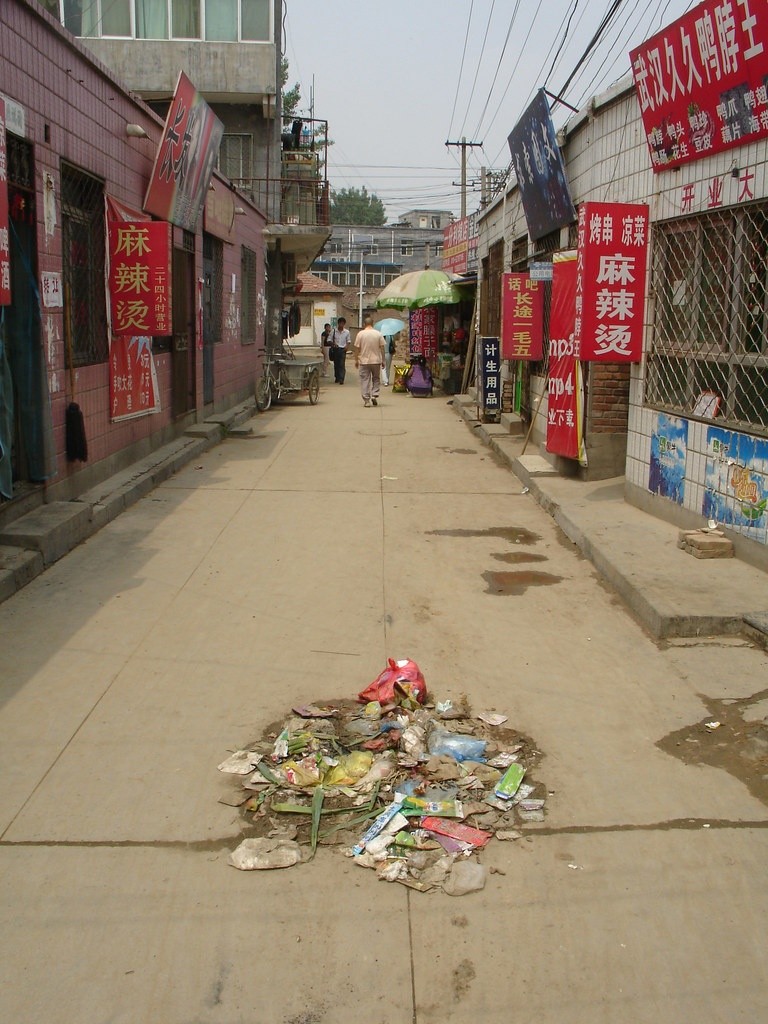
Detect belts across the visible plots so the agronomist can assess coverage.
[338,347,345,349]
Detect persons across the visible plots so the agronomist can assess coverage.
[321,323,330,367]
[326,317,351,385]
[403,355,434,398]
[178,106,219,208]
[354,318,386,408]
[380,335,396,387]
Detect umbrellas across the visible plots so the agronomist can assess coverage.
[373,318,406,337]
[373,270,475,374]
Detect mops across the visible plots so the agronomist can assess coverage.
[65,283,89,463]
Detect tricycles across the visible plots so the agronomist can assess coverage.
[254,346,326,413]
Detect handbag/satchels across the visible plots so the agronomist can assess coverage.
[389,335,396,354]
[329,348,335,361]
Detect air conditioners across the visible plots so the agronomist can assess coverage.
[285,259,298,284]
[338,257,348,262]
[331,257,338,262]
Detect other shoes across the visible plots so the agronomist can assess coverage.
[364,402,371,407]
[384,383,389,386]
[335,377,339,383]
[371,397,378,405]
[406,392,414,398]
[426,392,432,397]
[324,374,330,378]
[340,379,343,384]
